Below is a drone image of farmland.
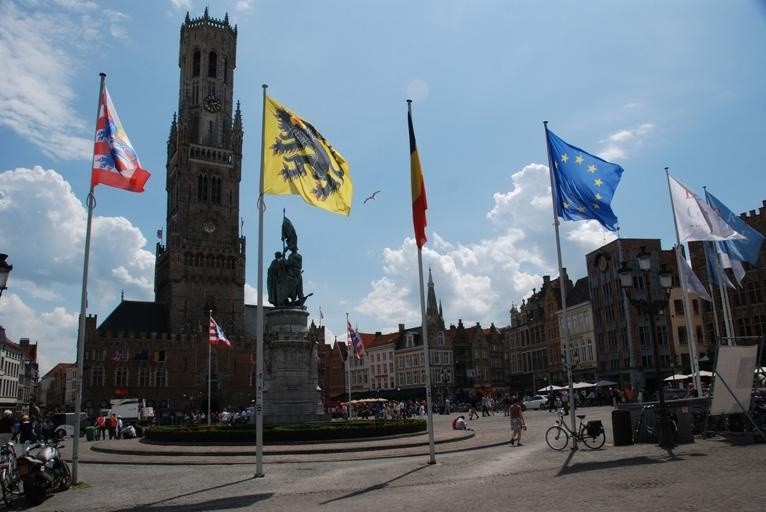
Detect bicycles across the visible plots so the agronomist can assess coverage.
[633,404,678,444]
[93,424,100,440]
[545,407,605,451]
[0,433,24,507]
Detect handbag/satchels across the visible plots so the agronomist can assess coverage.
[587,420,604,437]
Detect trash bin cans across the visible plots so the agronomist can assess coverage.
[84,425,96,440]
[611,409,631,446]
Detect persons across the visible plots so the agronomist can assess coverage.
[509,397,526,445]
[267,218,305,306]
[432,392,520,419]
[685,382,697,398]
[453,415,467,430]
[325,399,428,419]
[184,407,252,425]
[13,403,136,445]
[539,386,635,415]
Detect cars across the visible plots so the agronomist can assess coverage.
[47,413,89,439]
[521,395,562,411]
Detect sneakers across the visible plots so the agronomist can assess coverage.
[511,439,522,446]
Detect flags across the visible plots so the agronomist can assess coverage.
[667,174,764,302]
[348,322,363,359]
[263,95,353,216]
[92,79,151,192]
[408,112,427,250]
[547,129,624,232]
[208,317,231,347]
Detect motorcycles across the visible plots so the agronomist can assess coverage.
[15,435,72,505]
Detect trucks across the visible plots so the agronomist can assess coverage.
[98,398,139,428]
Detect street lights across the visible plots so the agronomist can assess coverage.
[617,244,673,449]
